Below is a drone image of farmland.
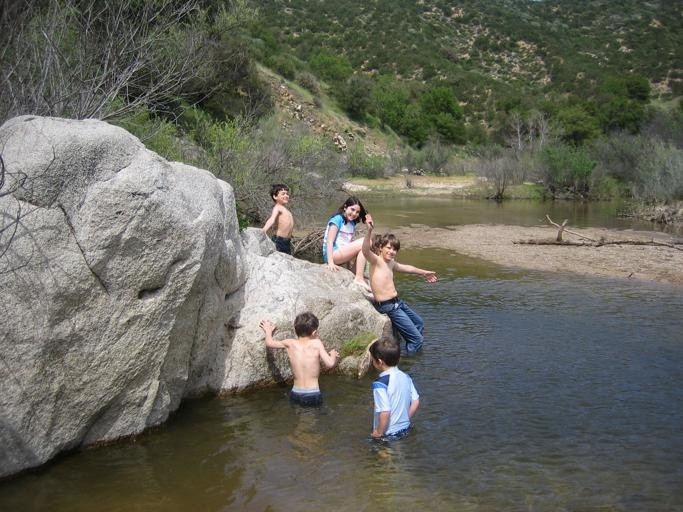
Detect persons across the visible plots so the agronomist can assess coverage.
[260,183,295,256]
[364,335,421,442]
[257,310,340,413]
[362,213,439,358]
[320,195,374,295]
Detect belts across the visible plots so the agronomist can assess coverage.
[376,296,399,306]
